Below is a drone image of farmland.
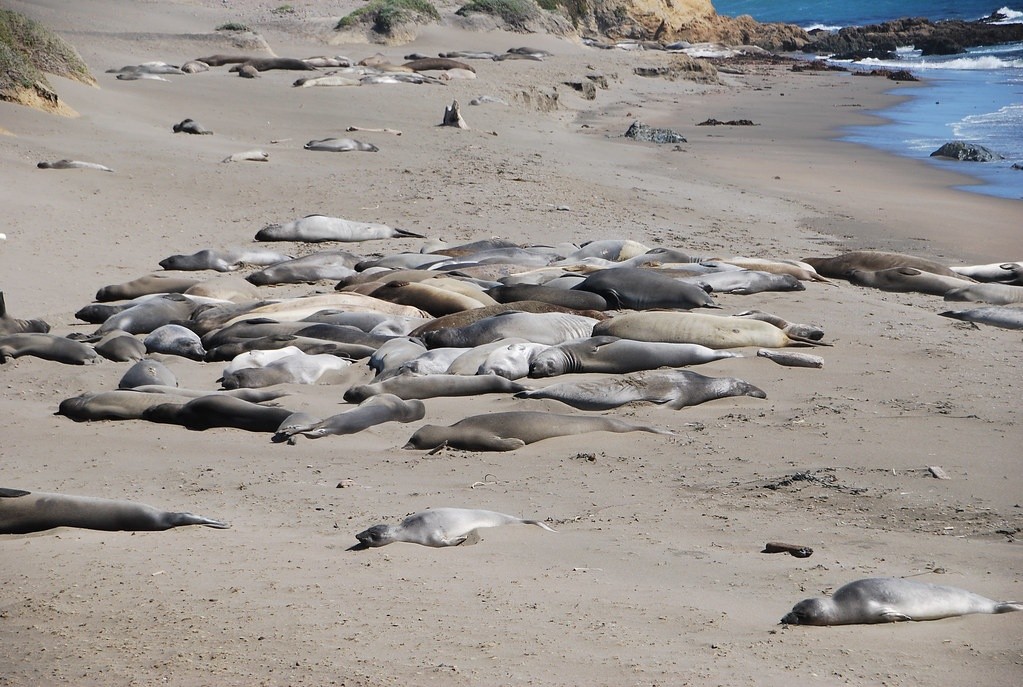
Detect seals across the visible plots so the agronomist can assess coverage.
[37,160,115,171]
[241,149,271,161]
[0,237,1023,452]
[105,46,558,87]
[346,126,402,136]
[172,119,214,135]
[781,578,1023,626]
[305,138,378,152]
[253,215,427,241]
[1,487,232,534]
[355,508,558,548]
